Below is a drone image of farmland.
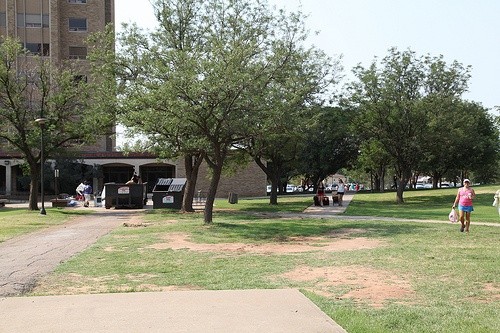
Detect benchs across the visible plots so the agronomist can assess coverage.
[50,199,71,207]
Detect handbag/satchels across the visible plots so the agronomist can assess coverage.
[448,209,460,223]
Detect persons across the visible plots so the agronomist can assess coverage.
[337,179,346,206]
[132,175,142,184]
[452,179,475,232]
[494,190,500,215]
[346,183,364,191]
[317,180,325,206]
[76,180,92,207]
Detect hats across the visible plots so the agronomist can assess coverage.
[463,179,471,184]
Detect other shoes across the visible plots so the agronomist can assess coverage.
[460,225,466,232]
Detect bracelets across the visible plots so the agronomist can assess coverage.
[454,204,456,206]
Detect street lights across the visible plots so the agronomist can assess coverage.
[35,117,50,215]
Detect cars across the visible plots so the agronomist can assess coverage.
[267,184,363,193]
[412,181,450,189]
